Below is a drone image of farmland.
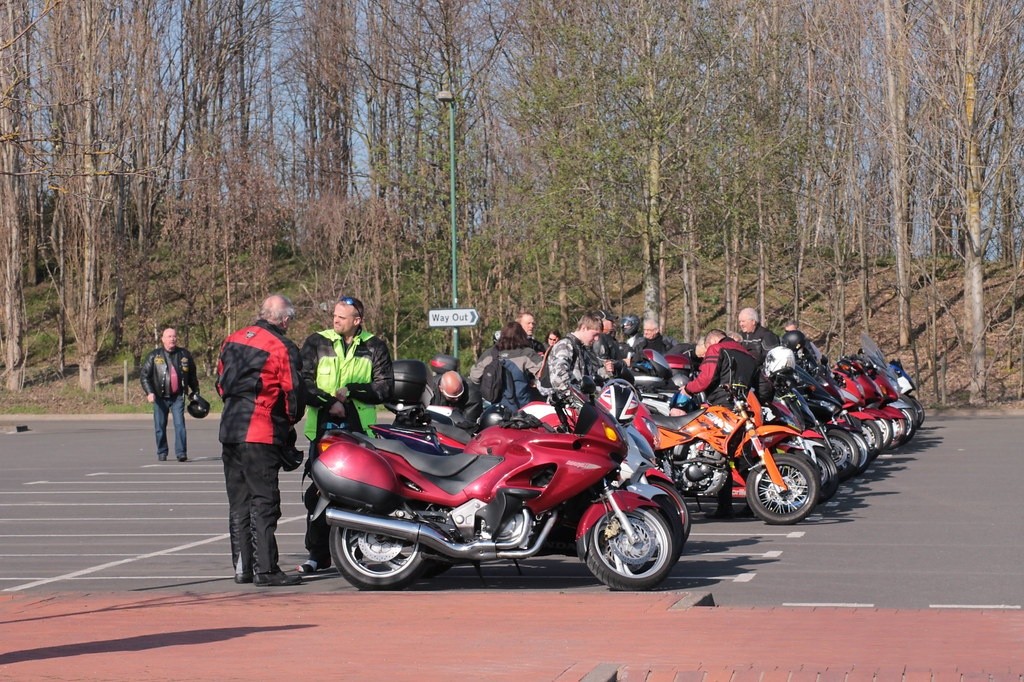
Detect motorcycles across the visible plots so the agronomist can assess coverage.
[312,329,924,590]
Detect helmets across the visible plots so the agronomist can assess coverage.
[633,349,673,379]
[669,389,700,413]
[479,403,511,432]
[764,345,796,377]
[440,370,465,398]
[781,330,806,352]
[187,392,210,419]
[598,379,640,427]
[620,314,640,335]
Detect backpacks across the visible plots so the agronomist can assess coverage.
[535,336,577,396]
[479,348,515,403]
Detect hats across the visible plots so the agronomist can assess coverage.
[597,309,619,322]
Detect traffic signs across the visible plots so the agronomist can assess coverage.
[429,309,481,327]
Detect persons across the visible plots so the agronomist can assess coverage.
[296,297,395,574]
[140,327,200,462]
[427,307,821,422]
[215,296,305,587]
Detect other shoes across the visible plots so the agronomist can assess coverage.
[234,571,254,584]
[254,566,302,587]
[295,558,332,572]
[159,455,166,461]
[177,454,187,461]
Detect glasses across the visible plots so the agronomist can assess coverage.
[549,337,556,341]
[340,296,362,316]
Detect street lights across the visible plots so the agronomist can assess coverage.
[437,90,457,359]
